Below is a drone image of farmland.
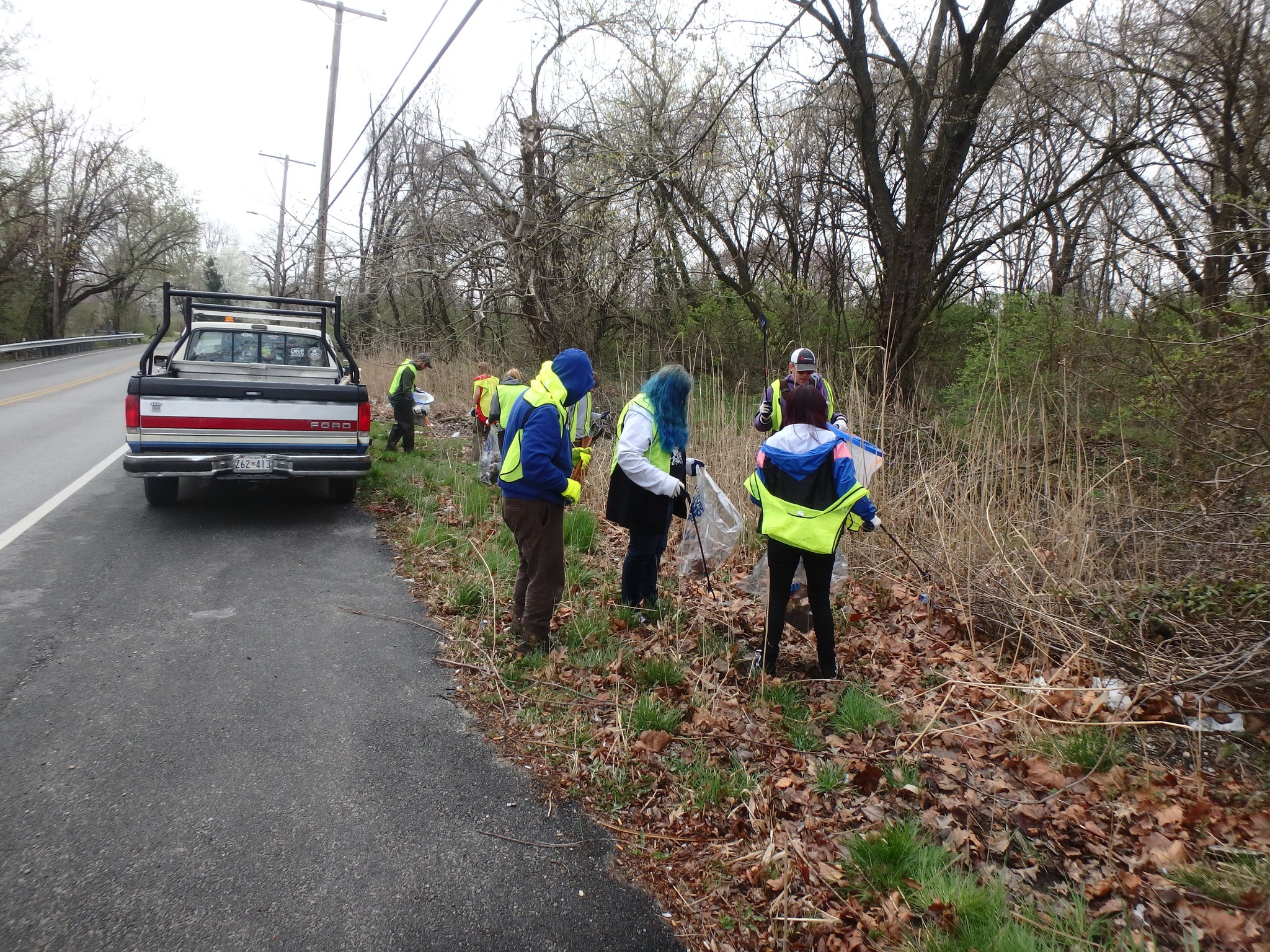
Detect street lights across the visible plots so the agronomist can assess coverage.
[246,210,285,324]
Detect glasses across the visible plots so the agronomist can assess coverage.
[798,370,814,374]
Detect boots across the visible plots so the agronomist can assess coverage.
[509,612,523,638]
[519,627,557,655]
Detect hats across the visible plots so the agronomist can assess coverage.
[418,353,432,369]
[790,348,817,372]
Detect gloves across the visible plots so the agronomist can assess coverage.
[863,515,882,532]
[601,411,612,422]
[672,481,684,498]
[414,405,422,415]
[413,386,422,393]
[561,477,581,506]
[834,415,848,432]
[470,406,477,417]
[760,401,773,419]
[685,458,705,476]
[571,446,592,470]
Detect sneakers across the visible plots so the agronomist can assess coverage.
[830,662,840,681]
[755,656,774,680]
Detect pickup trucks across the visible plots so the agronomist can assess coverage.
[121,281,372,504]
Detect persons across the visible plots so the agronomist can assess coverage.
[605,363,706,609]
[498,347,595,658]
[756,347,850,435]
[485,369,529,460]
[565,371,611,493]
[384,353,433,455]
[471,362,500,467]
[743,384,882,679]
